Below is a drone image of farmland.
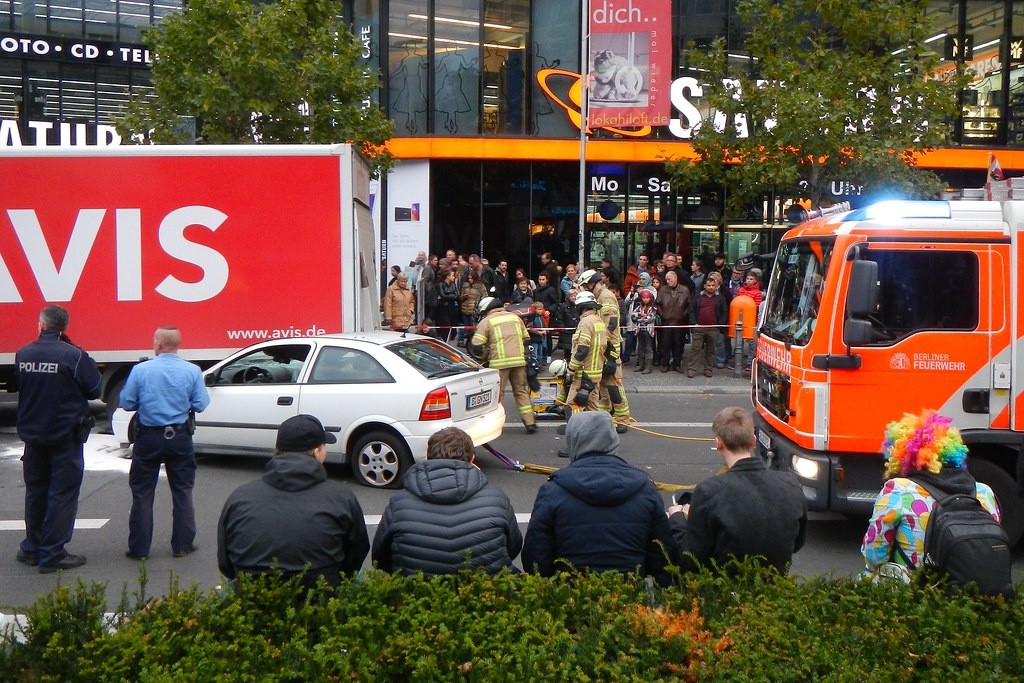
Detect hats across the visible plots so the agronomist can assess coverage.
[275,413,337,452]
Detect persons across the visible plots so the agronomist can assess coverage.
[217,414,371,598]
[601,251,763,377]
[594,51,637,100]
[859,408,1001,584]
[549,270,629,457]
[384,250,580,372]
[120,326,210,560]
[6,305,102,573]
[521,410,672,590]
[471,297,538,432]
[700,245,716,256]
[666,406,808,585]
[793,273,823,315]
[372,426,523,582]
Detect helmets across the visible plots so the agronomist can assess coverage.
[550,358,569,378]
[577,270,603,286]
[476,296,503,315]
[573,291,602,312]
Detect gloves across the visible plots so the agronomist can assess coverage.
[605,359,617,377]
[526,366,541,391]
[557,405,566,415]
[548,404,558,413]
[572,379,594,407]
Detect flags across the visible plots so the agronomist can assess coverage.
[589,0,672,128]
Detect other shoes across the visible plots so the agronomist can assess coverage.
[717,361,725,369]
[622,361,630,365]
[636,362,639,368]
[558,450,571,457]
[36,554,88,574]
[124,550,150,561]
[725,362,735,370]
[746,365,752,371]
[703,369,712,378]
[654,361,660,366]
[170,544,197,558]
[16,549,41,566]
[526,424,541,433]
[686,370,695,378]
[661,366,670,373]
[643,369,652,375]
[617,425,630,433]
[674,366,684,373]
[633,366,644,372]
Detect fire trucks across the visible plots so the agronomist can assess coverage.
[734,175,1024,558]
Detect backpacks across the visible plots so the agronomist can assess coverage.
[895,475,1017,600]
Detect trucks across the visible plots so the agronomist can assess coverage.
[1,140,380,429]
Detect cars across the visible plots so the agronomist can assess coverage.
[110,329,506,490]
